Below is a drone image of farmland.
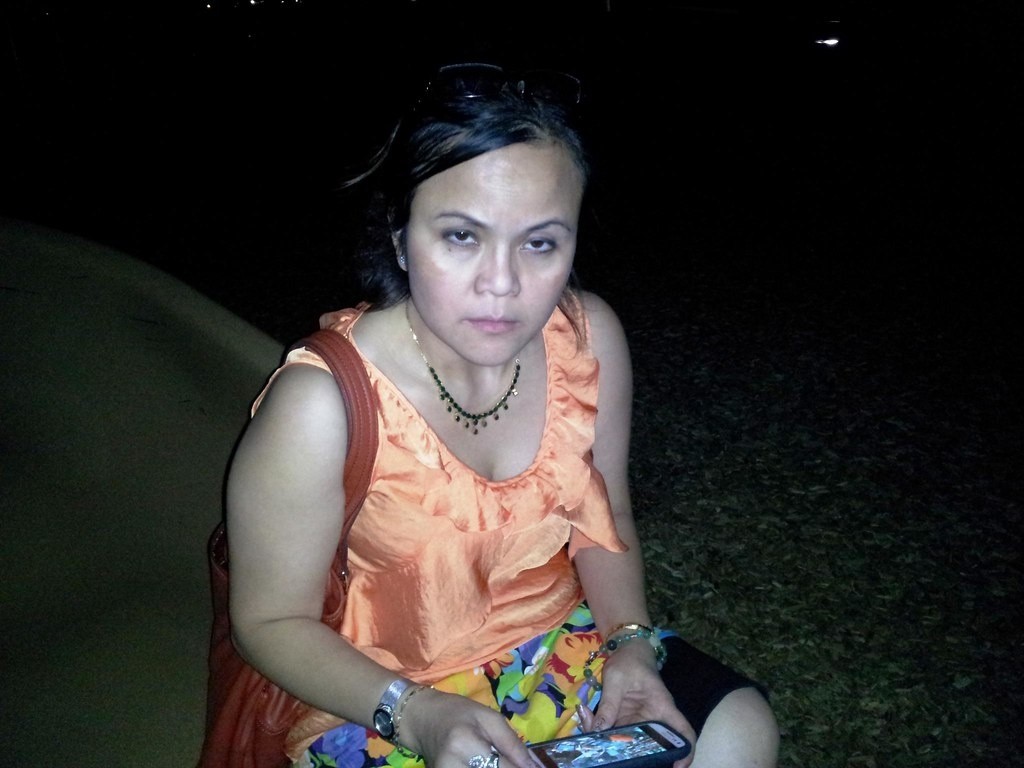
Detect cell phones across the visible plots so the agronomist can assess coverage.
[528,720,691,768]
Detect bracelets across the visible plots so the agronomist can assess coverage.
[584,623,667,691]
[396,686,435,758]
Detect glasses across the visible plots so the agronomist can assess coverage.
[405,62,588,130]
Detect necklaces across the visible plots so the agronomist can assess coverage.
[405,306,522,435]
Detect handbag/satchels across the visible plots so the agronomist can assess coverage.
[194,329,379,768]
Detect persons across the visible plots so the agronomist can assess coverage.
[225,87,780,768]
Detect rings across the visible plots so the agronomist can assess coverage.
[468,753,499,768]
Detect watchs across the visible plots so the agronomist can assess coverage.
[373,678,416,742]
